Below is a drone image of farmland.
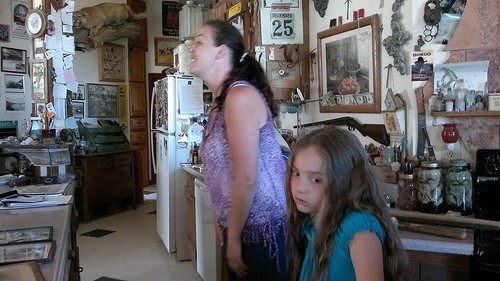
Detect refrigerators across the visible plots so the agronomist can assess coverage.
[151,76,204,254]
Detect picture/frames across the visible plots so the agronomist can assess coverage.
[4,74,25,93]
[5,96,26,112]
[25,5,47,38]
[317,14,380,114]
[72,101,84,118]
[0,24,10,42]
[0,46,27,74]
[70,84,85,100]
[85,82,119,118]
[154,1,183,66]
[0,226,53,245]
[0,261,46,281]
[0,240,57,266]
[30,59,48,103]
[98,41,125,82]
[382,113,400,134]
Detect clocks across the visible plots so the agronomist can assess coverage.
[0,151,20,176]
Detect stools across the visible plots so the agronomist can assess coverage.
[143,184,156,214]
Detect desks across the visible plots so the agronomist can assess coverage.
[0,144,83,281]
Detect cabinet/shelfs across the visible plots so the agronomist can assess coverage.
[185,173,195,270]
[407,250,472,281]
[76,0,149,224]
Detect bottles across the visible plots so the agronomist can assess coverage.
[428,87,500,112]
[397,163,418,211]
[352,9,365,20]
[442,123,458,143]
[190,142,201,165]
[329,16,343,28]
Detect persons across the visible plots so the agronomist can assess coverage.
[189,20,299,281]
[288,125,408,281]
